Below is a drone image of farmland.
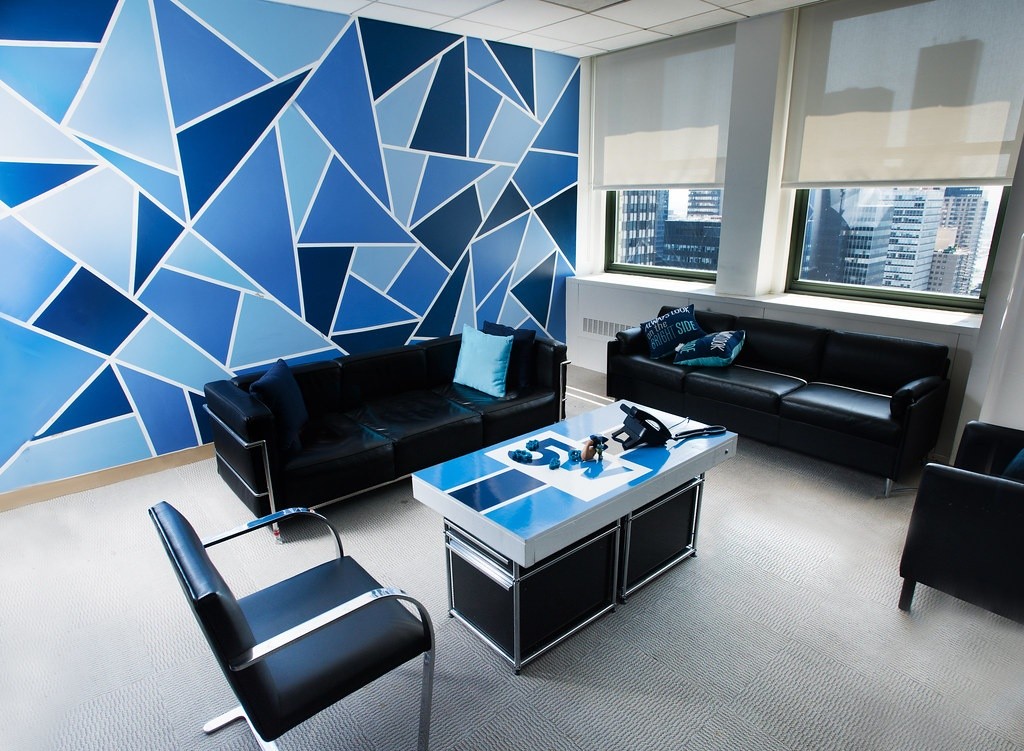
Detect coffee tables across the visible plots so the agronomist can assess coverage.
[412,399,738,675]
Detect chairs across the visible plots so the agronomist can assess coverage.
[148,501,436,751]
[898,420,1024,625]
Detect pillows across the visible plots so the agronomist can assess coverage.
[672,330,746,368]
[640,304,707,361]
[249,359,310,445]
[452,320,537,400]
[1001,449,1024,486]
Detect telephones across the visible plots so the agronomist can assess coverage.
[611,403,673,450]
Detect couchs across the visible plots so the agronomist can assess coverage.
[606,306,953,501]
[203,330,572,547]
